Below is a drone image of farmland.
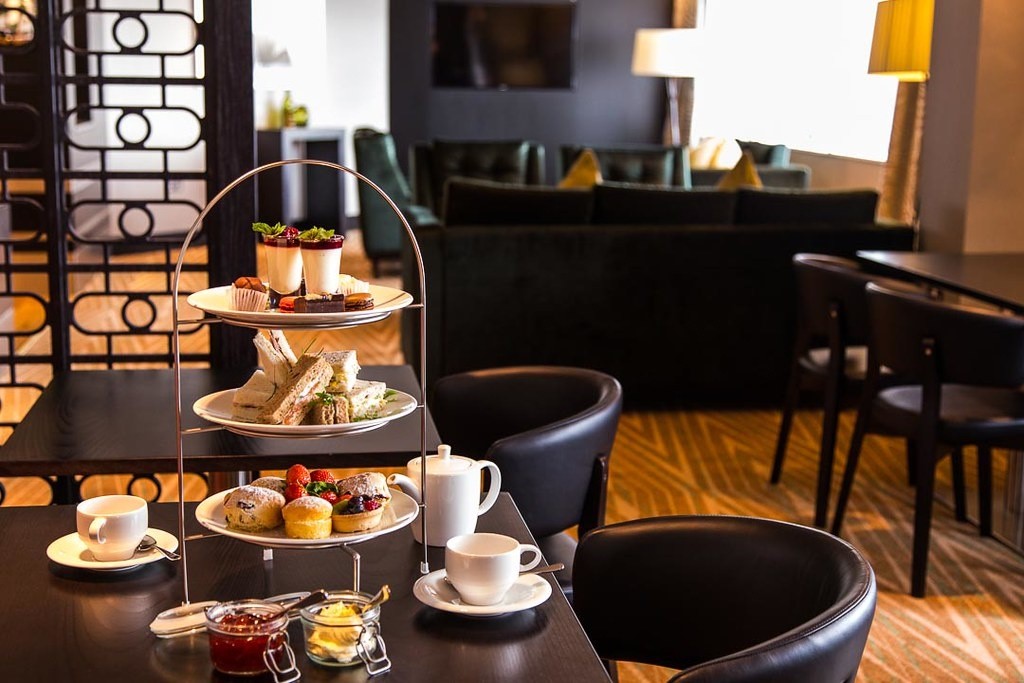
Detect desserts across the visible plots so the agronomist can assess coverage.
[219,463,393,540]
[232,215,378,315]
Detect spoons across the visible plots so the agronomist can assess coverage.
[136,535,182,562]
[444,563,564,585]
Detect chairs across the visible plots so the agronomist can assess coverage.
[428,365,623,609]
[831,280,1024,599]
[573,514,877,683]
[770,252,969,529]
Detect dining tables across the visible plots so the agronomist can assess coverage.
[0,365,444,501]
[856,249,1024,318]
[1,491,614,683]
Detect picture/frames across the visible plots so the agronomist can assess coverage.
[427,0,578,93]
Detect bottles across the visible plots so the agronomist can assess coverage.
[267,90,296,130]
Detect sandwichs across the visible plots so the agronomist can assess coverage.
[224,328,386,425]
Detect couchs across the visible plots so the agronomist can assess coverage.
[352,125,919,416]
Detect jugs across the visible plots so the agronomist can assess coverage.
[386,444,501,547]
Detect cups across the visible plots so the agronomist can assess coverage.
[445,533,542,605]
[204,599,289,676]
[299,590,381,666]
[77,495,148,560]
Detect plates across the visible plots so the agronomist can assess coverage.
[187,283,414,330]
[412,568,553,616]
[195,486,419,548]
[46,528,179,571]
[192,387,417,438]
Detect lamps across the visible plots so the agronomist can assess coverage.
[630,28,719,147]
[867,0,936,231]
